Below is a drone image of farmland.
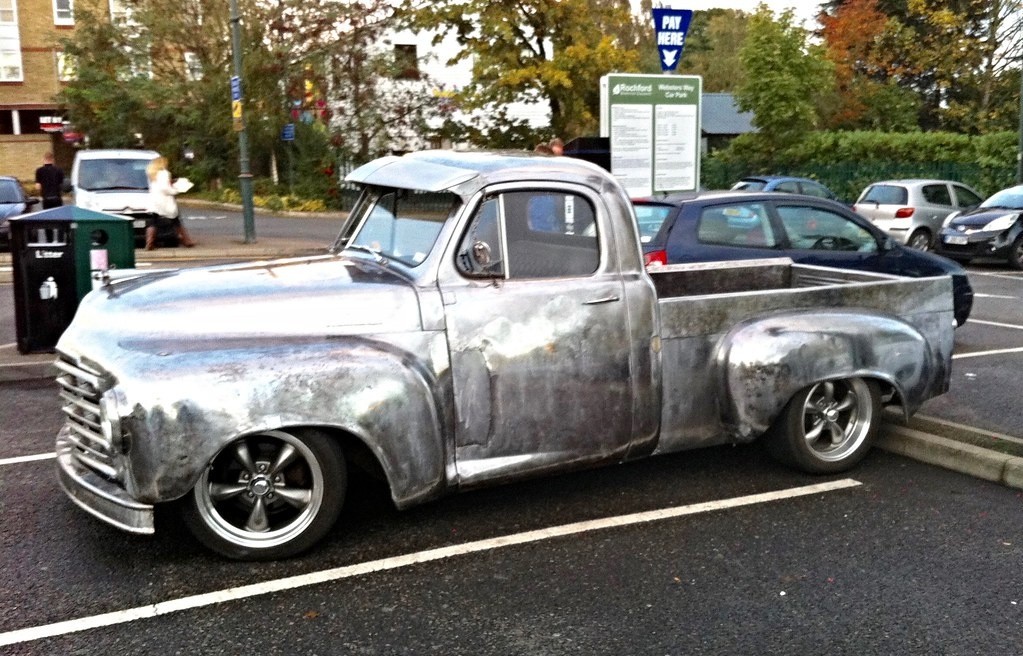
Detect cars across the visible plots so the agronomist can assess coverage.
[939,182,1022,275]
[724,175,849,235]
[0,175,40,251]
[60,147,180,248]
[849,178,986,260]
[578,188,975,338]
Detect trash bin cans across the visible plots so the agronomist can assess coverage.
[10,206,134,354]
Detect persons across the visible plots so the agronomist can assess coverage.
[35,152,67,242]
[142,156,196,251]
[526,137,564,233]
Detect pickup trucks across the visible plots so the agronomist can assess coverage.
[48,146,961,562]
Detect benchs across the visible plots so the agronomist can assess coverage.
[509,240,598,279]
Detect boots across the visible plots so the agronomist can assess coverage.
[176,226,197,247]
[144,227,157,249]
